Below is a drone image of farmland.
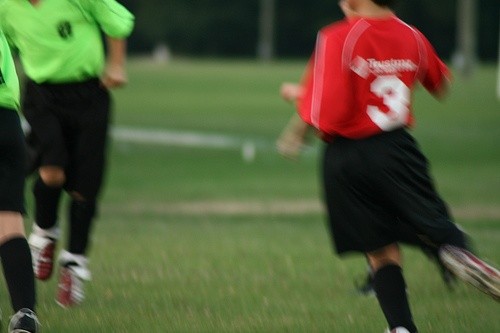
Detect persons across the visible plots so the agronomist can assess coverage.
[0,0,135,333]
[268,0,500,333]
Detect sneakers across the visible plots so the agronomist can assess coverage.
[28,233,57,281]
[438,245,500,302]
[54,265,92,311]
[8,307,39,333]
